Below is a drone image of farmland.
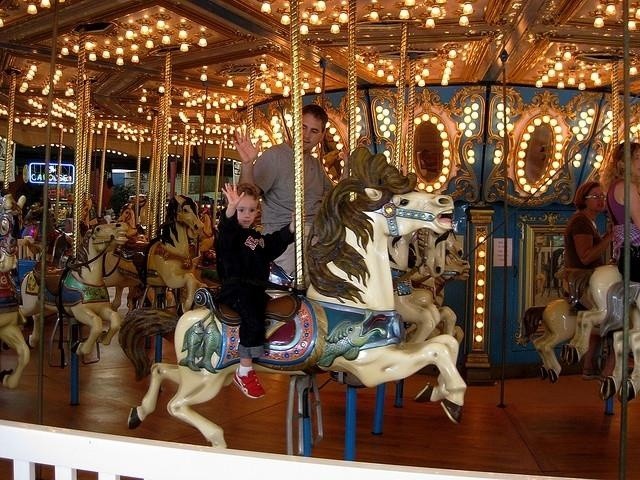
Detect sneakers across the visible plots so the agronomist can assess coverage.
[232,366,266,400]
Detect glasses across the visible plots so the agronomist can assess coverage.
[584,193,609,202]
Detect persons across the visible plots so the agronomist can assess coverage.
[563,182,615,309]
[215,182,296,400]
[231,105,337,285]
[606,142,639,285]
